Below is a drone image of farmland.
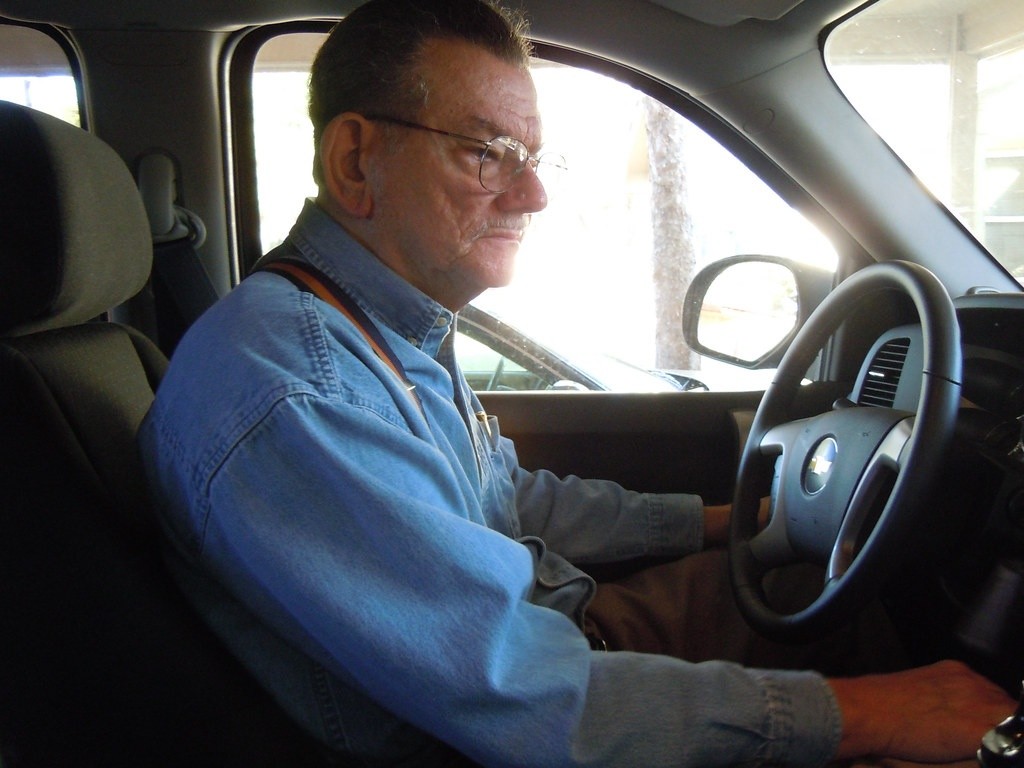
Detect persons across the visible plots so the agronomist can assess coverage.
[131,0,1019,768]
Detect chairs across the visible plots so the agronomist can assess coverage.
[0,98,171,768]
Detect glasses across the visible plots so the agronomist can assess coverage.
[362,110,568,203]
